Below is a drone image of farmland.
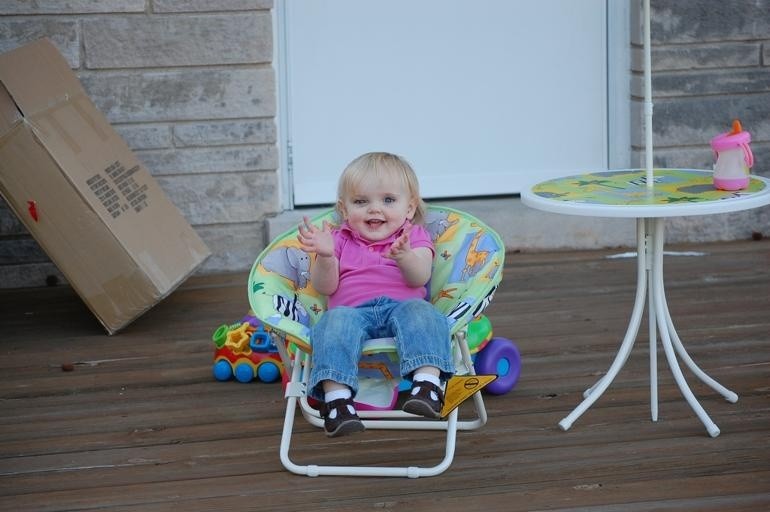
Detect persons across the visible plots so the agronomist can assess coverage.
[296,151,455,437]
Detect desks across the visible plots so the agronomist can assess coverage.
[517,167,770,439]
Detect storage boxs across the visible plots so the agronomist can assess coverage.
[0,38,212,334]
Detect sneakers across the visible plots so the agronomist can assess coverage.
[403,380,444,418]
[320,397,364,437]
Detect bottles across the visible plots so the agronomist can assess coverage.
[711,118,754,191]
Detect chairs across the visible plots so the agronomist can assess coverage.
[246,199,504,479]
